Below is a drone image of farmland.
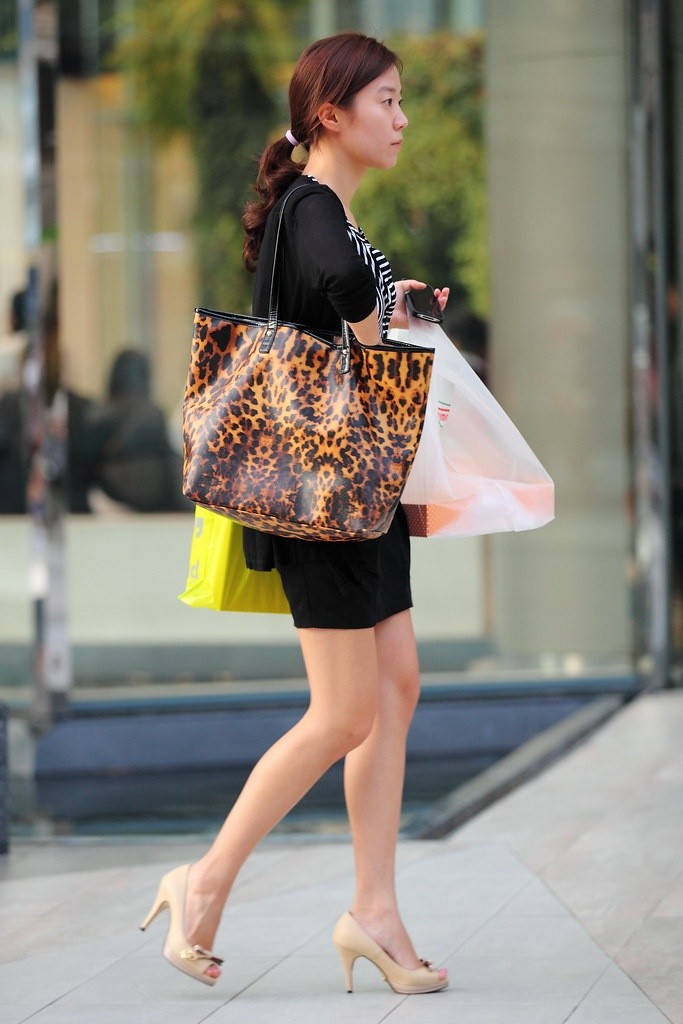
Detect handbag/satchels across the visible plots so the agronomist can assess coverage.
[381,291,556,539]
[175,181,436,540]
[177,503,294,614]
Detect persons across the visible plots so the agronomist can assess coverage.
[140,34,452,997]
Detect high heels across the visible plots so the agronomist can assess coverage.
[334,909,451,996]
[139,862,223,988]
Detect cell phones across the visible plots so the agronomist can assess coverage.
[405,284,444,324]
